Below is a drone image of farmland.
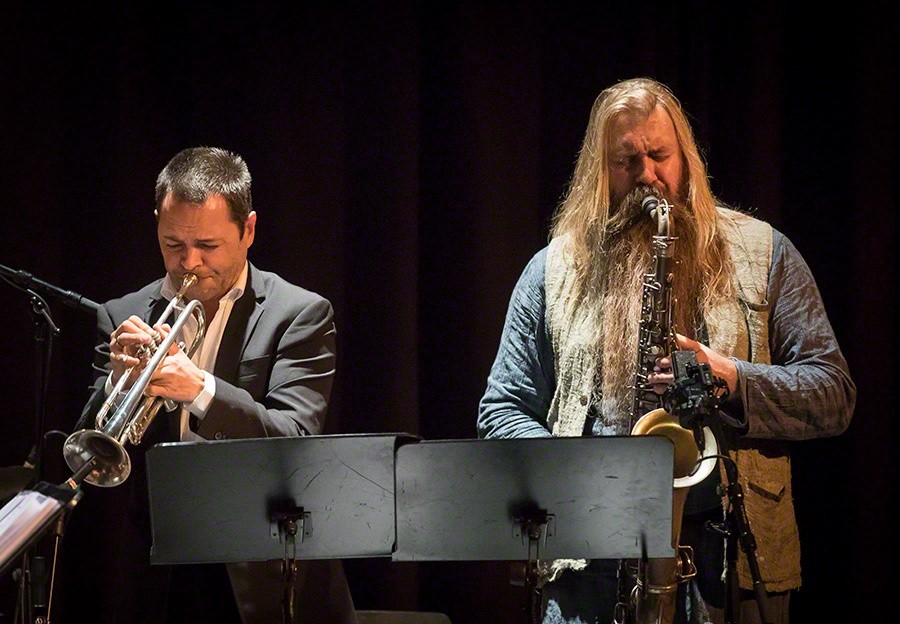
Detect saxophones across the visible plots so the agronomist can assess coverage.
[611,195,718,624]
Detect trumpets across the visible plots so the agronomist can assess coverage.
[63,272,207,487]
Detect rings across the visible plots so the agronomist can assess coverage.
[116,336,124,348]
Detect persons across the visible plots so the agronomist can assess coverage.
[73,146,336,624]
[477,78,855,623]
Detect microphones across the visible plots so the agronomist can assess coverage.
[670,350,711,429]
